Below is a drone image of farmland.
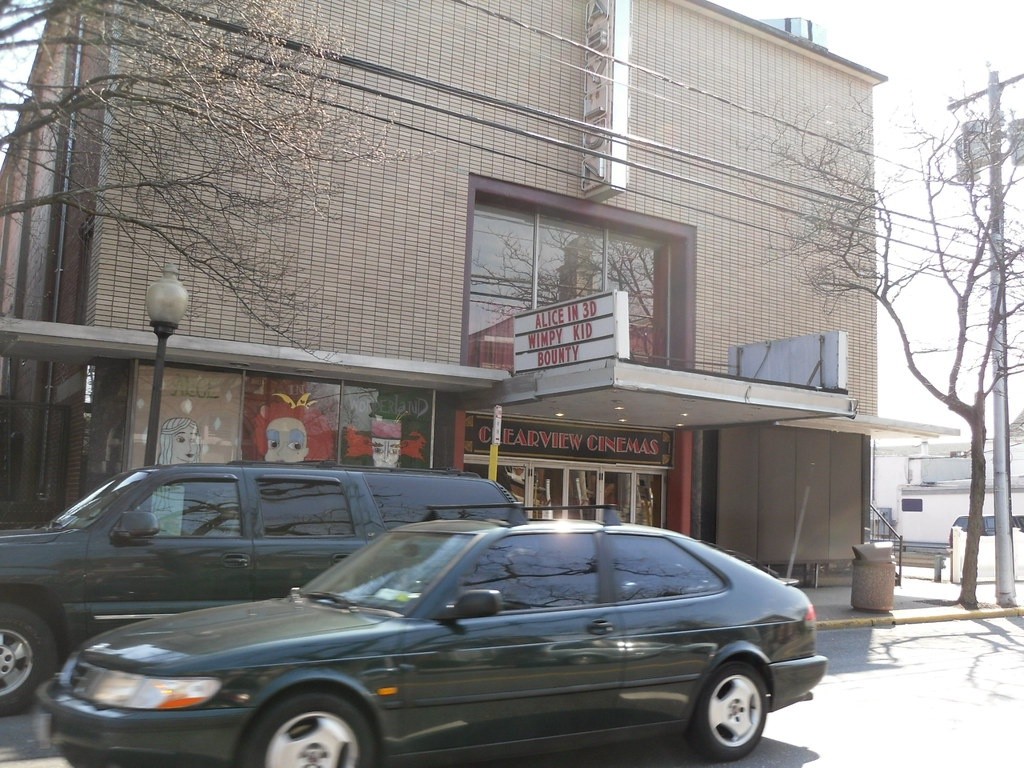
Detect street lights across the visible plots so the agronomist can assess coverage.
[140,258,190,513]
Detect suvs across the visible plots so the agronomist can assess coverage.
[1,459,526,718]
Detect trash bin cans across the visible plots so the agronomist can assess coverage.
[850,541,896,613]
[1012,515,1024,582]
[949,516,996,584]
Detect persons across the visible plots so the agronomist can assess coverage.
[161,498,241,539]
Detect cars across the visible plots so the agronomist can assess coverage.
[32,500,829,768]
